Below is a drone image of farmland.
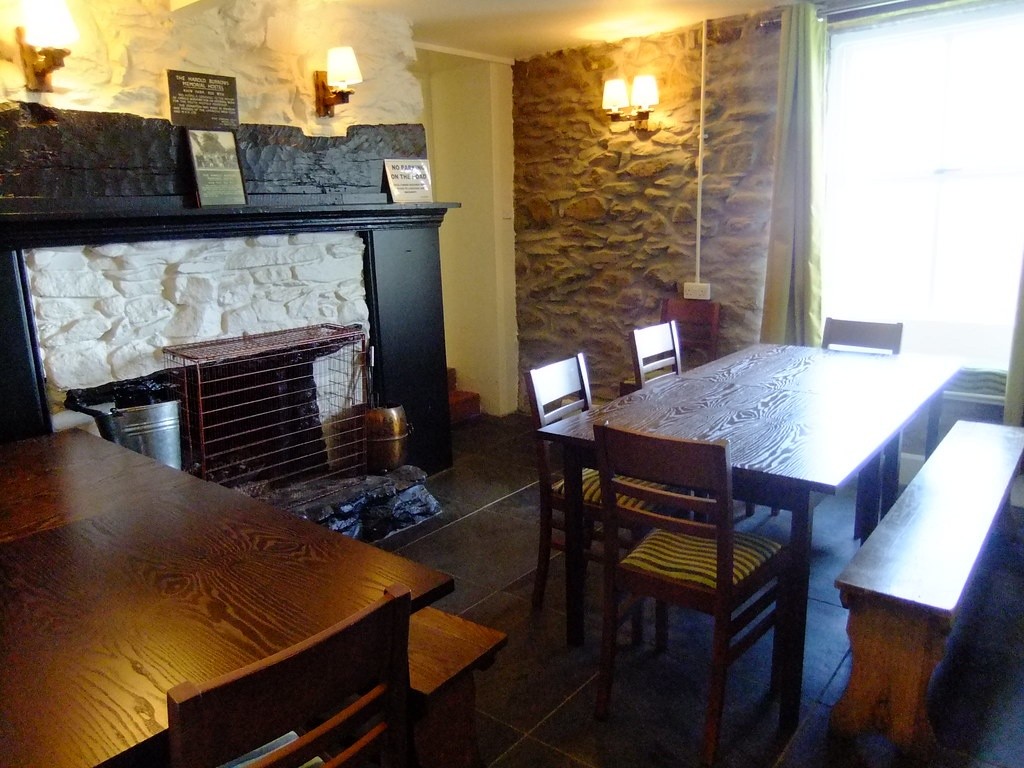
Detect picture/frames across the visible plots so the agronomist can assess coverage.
[185,126,251,208]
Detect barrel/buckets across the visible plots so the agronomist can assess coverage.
[108,400,184,472]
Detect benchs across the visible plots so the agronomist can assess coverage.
[833,419,1023,752]
[409,605,509,703]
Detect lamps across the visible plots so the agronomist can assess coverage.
[602,74,660,121]
[313,45,363,118]
[14,0,79,92]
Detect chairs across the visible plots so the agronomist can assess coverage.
[591,419,797,768]
[770,318,903,516]
[164,584,420,768]
[520,350,690,642]
[621,318,755,516]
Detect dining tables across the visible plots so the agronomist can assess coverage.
[537,343,967,713]
[0,426,457,768]
